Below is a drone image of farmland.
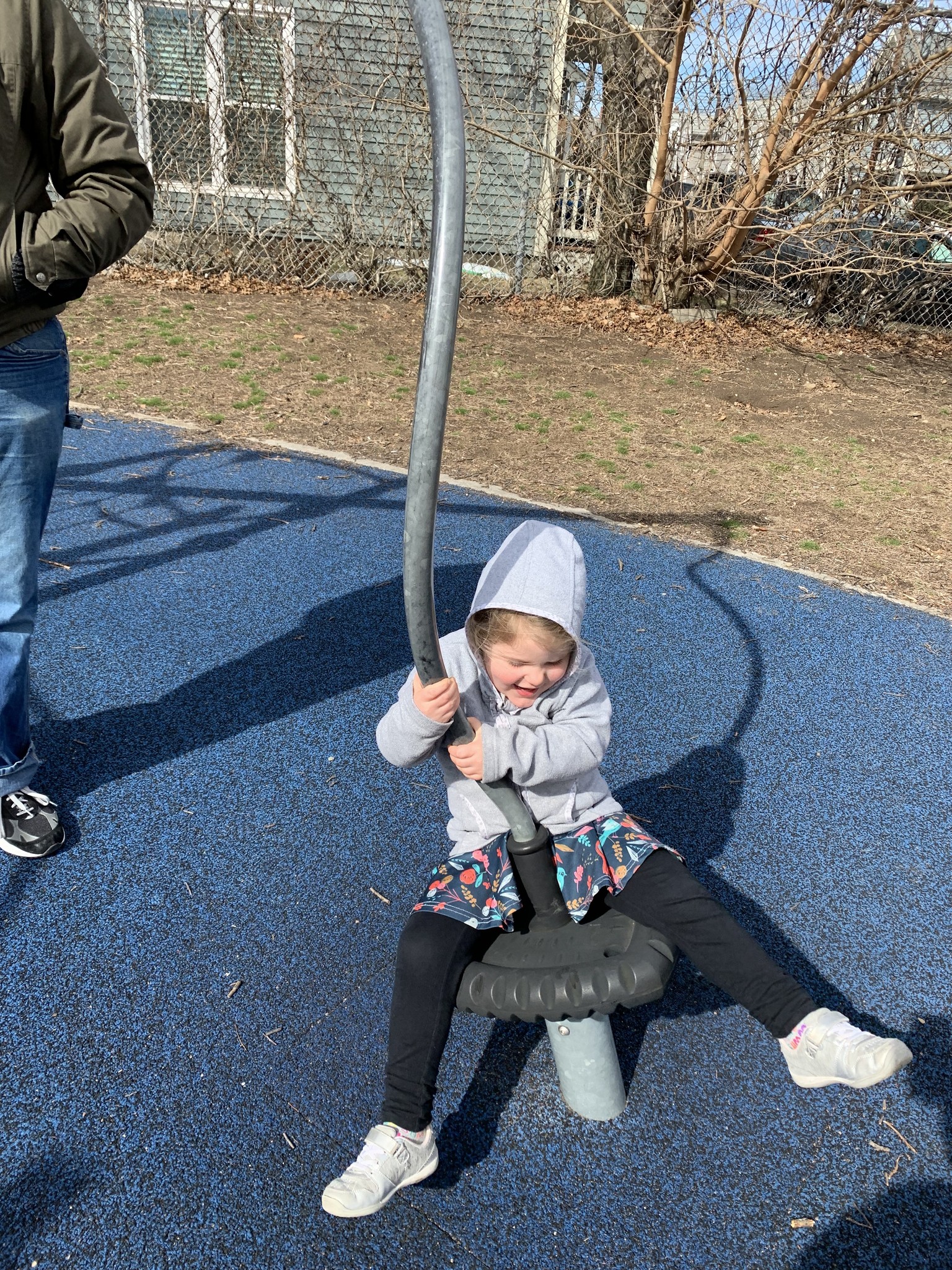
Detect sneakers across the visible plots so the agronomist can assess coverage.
[321,1123,439,1217]
[0,786,66,857]
[774,1006,912,1089]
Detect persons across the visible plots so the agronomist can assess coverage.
[320,519,914,1219]
[0,0,157,859]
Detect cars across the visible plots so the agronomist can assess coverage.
[695,171,952,327]
